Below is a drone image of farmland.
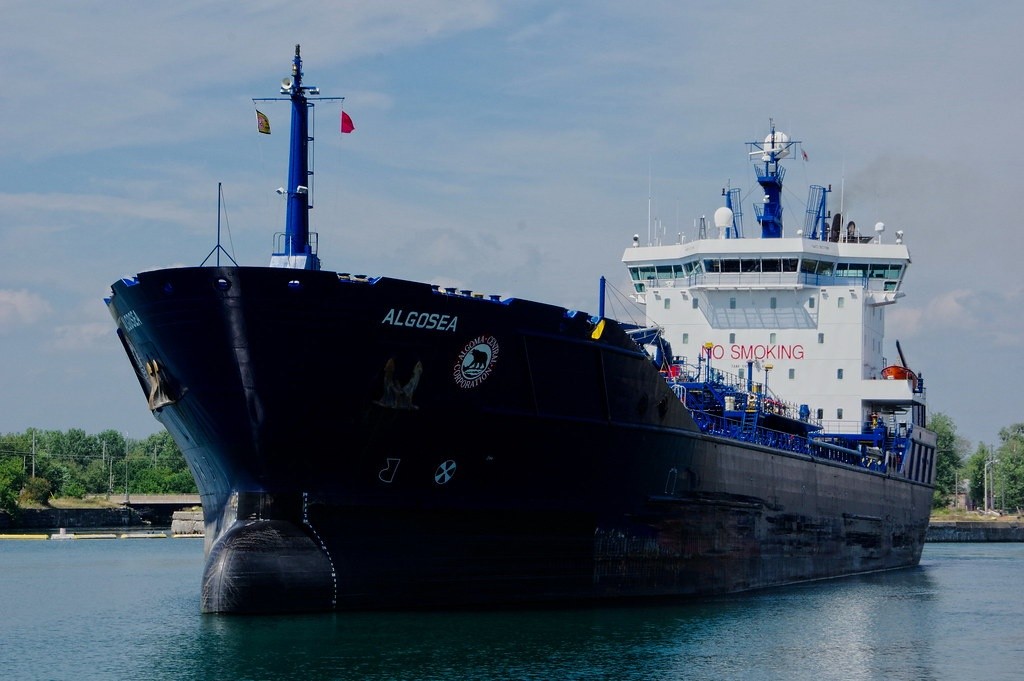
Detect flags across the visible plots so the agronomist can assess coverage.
[341,111,355,133]
[257,110,272,134]
[802,149,808,160]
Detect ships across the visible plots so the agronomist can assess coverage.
[101,40,938,629]
